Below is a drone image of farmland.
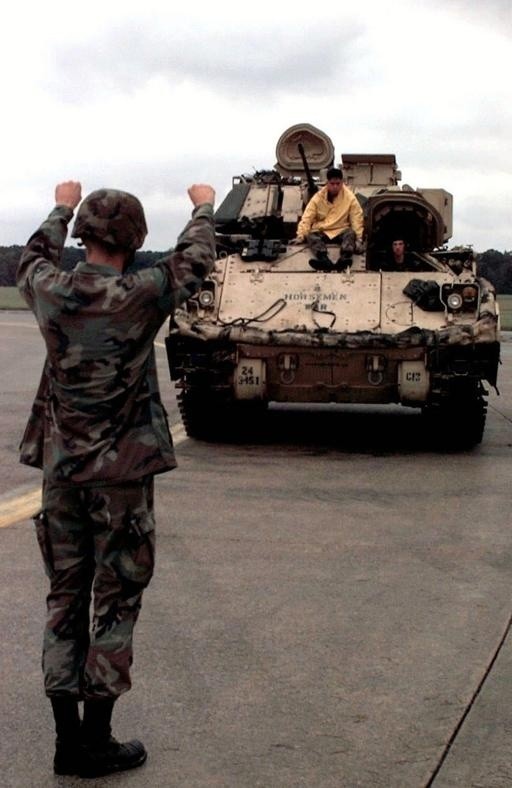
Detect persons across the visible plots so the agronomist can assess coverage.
[17,180,214,780]
[379,238,418,271]
[288,168,365,274]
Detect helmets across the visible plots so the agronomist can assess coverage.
[70,189,147,248]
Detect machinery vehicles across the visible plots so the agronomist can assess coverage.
[162,123,504,452]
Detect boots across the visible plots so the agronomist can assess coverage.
[51,696,84,775]
[79,698,147,777]
[336,250,352,270]
[309,252,335,271]
